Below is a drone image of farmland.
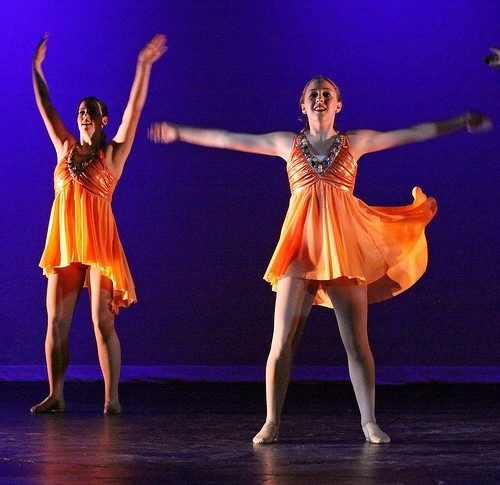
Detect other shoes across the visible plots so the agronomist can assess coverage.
[362,421,390,443]
[30,395,64,413]
[103,399,121,415]
[252,423,279,444]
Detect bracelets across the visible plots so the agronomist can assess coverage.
[175,126,180,141]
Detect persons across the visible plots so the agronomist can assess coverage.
[147,77,492,444]
[483,47,500,66]
[31,31,167,414]
